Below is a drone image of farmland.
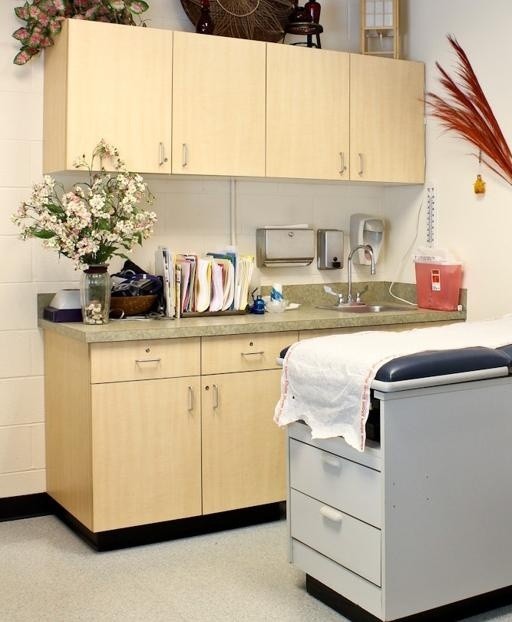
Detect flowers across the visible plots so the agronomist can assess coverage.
[9,0,150,64]
[7,138,161,285]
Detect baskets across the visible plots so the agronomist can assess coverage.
[110,295,160,315]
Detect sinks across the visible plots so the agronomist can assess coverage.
[316,304,417,313]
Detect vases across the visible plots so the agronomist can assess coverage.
[78,269,112,324]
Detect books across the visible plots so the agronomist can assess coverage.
[155,249,255,318]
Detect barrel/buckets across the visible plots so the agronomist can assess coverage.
[415,255,463,312]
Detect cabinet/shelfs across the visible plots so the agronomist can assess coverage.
[37,330,297,553]
[276,335,512,620]
[266,32,425,187]
[45,17,265,188]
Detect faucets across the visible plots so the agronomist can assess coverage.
[347,245,377,302]
